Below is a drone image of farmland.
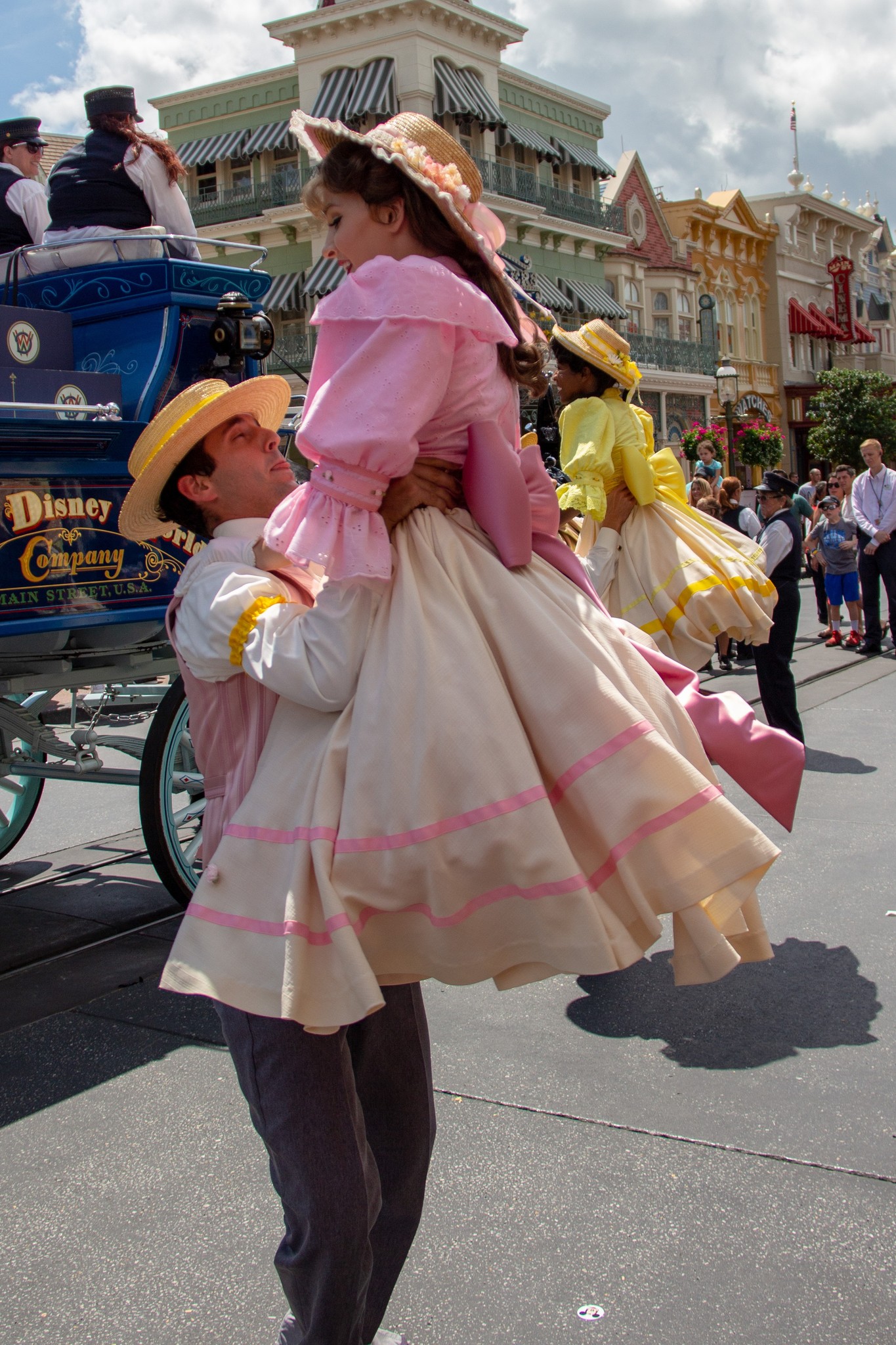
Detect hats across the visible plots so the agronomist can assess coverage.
[0,117,49,146]
[694,466,713,478]
[83,85,144,123]
[118,375,291,539]
[287,108,506,279]
[753,471,798,498]
[552,318,643,390]
[818,495,841,508]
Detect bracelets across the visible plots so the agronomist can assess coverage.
[812,550,818,555]
[811,548,817,553]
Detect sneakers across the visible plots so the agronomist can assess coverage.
[825,630,842,646]
[845,630,860,646]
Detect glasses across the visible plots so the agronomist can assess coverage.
[755,493,780,501]
[793,476,798,478]
[827,482,840,488]
[12,142,44,156]
[822,505,838,511]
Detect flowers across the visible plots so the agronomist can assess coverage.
[678,421,729,464]
[730,417,787,471]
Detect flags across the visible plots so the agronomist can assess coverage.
[790,101,796,130]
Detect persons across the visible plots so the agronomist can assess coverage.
[851,438,896,657]
[546,320,777,671]
[755,471,804,746]
[685,441,760,671]
[757,464,890,647]
[41,85,201,260]
[0,116,52,256]
[550,478,637,599]
[119,377,459,1345]
[159,107,805,1038]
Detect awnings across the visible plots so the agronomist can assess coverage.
[432,58,508,134]
[175,120,299,169]
[494,121,562,163]
[309,59,401,124]
[557,278,628,320]
[255,272,308,314]
[300,255,348,300]
[506,270,575,315]
[788,298,845,339]
[826,305,875,345]
[552,137,616,181]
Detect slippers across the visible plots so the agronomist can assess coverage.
[881,625,890,640]
[860,633,865,640]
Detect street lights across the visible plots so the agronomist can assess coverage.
[714,357,739,478]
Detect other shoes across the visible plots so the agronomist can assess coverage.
[697,659,713,671]
[819,615,844,624]
[817,628,833,638]
[737,653,755,660]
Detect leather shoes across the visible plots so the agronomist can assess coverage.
[718,650,737,660]
[855,642,882,653]
[719,657,732,670]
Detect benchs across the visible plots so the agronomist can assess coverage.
[0,225,274,327]
